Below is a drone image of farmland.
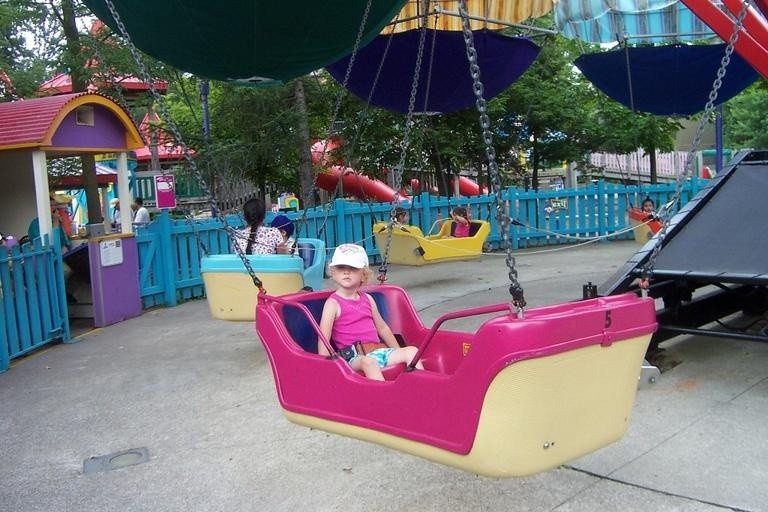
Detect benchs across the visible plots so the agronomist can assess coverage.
[443,220,483,239]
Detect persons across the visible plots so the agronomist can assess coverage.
[440,205,472,240]
[132,197,150,229]
[270,213,301,256]
[640,195,657,217]
[393,206,409,225]
[316,241,426,381]
[27,195,78,304]
[233,197,290,255]
[110,198,135,234]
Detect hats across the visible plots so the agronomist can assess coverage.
[330,244,369,269]
[271,216,294,235]
[109,198,119,208]
[390,207,406,217]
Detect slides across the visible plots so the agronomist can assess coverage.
[412,175,489,195]
[310,138,411,202]
